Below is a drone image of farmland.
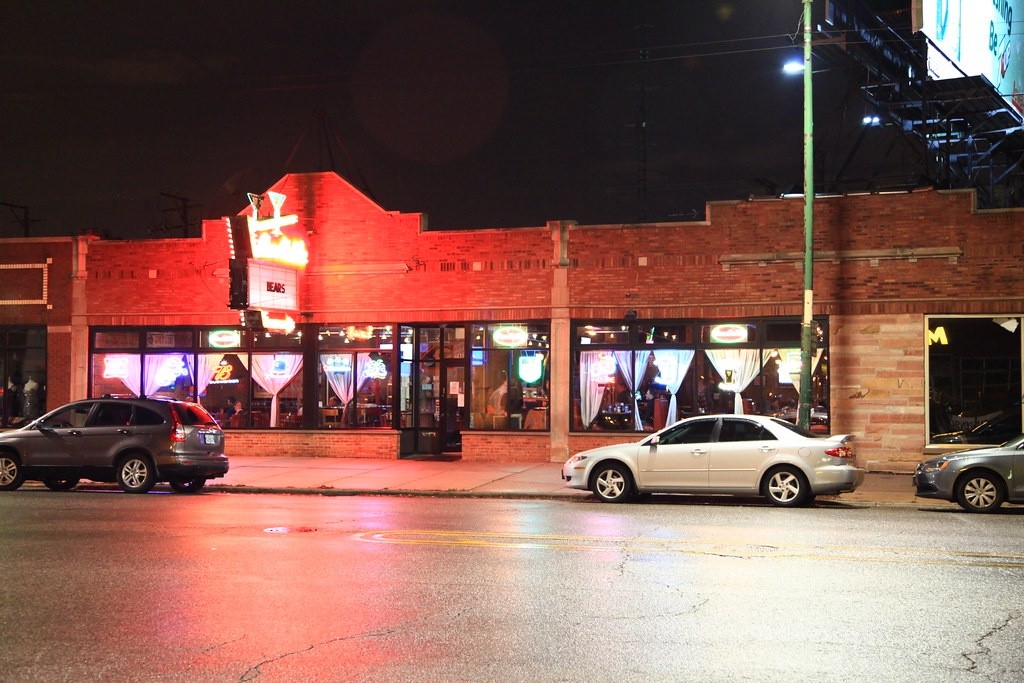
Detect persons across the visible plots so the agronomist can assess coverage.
[329,397,338,406]
[769,397,827,414]
[22,376,38,417]
[223,397,236,417]
[637,387,656,426]
[698,377,715,414]
[8,377,17,416]
[231,401,242,415]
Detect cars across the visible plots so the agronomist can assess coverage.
[912,433,1024,512]
[561,414,865,507]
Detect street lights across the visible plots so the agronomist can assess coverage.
[782,62,813,435]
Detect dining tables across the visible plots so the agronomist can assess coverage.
[602,409,632,430]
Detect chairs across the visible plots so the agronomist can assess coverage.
[643,398,668,432]
[573,399,603,432]
[216,413,303,428]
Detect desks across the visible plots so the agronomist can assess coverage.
[780,411,828,424]
[493,414,522,429]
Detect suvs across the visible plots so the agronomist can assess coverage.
[0,393,229,496]
